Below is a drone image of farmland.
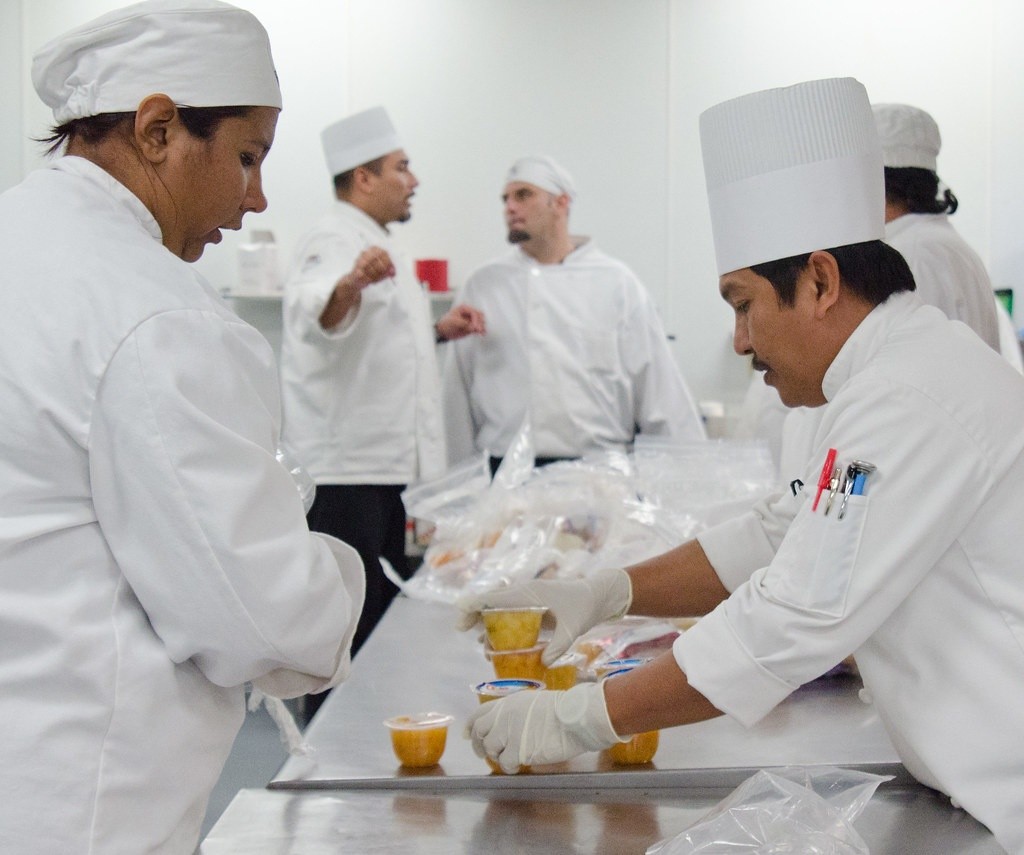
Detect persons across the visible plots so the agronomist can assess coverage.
[0,0,366,855]
[282,79,1024,855]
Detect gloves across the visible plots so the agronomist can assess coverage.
[461,677,635,775]
[452,566,632,668]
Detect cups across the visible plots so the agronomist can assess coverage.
[382,711,455,769]
[473,606,662,774]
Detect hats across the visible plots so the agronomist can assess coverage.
[699,77,885,278]
[30,0,283,128]
[502,157,577,202]
[319,104,403,178]
[868,103,942,171]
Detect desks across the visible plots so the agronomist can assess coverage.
[195,561,1009,855]
